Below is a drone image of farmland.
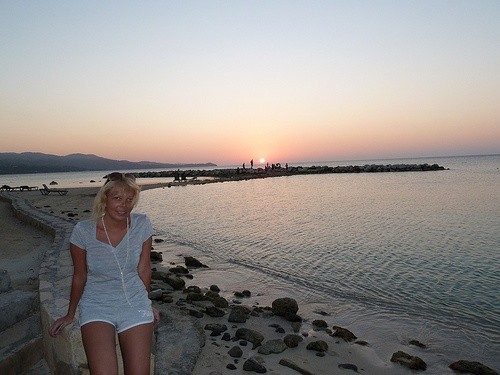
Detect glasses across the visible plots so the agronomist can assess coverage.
[103,172,136,187]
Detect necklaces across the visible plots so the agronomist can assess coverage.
[100,217,130,306]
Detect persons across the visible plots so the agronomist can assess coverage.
[251,159,253,169]
[265,162,288,169]
[243,163,245,169]
[49,172,161,375]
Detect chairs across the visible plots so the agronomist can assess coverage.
[0,184,70,196]
